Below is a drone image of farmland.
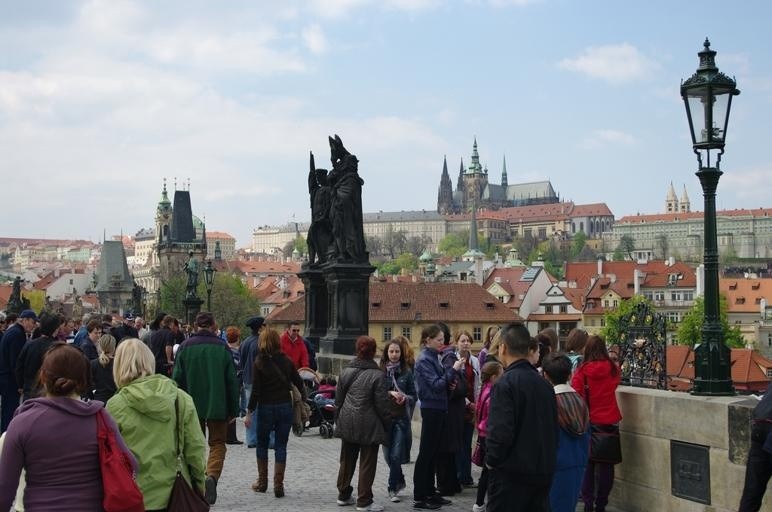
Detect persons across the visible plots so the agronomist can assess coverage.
[0,310,38,438]
[484,323,559,512]
[570,335,623,512]
[88,333,118,408]
[238,318,276,449]
[172,318,186,347]
[379,334,419,503]
[52,314,67,342]
[65,318,75,343]
[79,320,103,360]
[0,344,141,512]
[104,338,209,512]
[333,334,390,512]
[113,312,139,349]
[455,329,481,488]
[280,320,309,370]
[172,312,240,505]
[134,317,146,340]
[542,351,593,512]
[225,326,245,446]
[470,325,590,512]
[102,313,112,337]
[153,314,175,376]
[244,327,308,498]
[14,315,62,403]
[412,321,466,510]
[141,312,167,351]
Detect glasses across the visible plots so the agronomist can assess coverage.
[293,329,300,332]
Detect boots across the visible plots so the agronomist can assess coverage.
[252,458,269,493]
[274,462,286,498]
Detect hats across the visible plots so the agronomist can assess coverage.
[246,317,265,326]
[123,312,135,321]
[19,309,40,322]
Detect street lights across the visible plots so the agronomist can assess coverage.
[142,287,149,320]
[202,259,218,311]
[680,36,741,396]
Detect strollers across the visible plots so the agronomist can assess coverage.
[292,367,338,439]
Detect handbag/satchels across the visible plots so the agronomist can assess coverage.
[583,372,623,464]
[472,392,489,465]
[97,410,145,512]
[166,396,210,512]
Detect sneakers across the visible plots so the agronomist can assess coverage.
[413,501,441,510]
[388,492,400,502]
[205,477,217,504]
[356,503,384,511]
[398,484,407,490]
[337,495,357,505]
[473,503,485,512]
[226,440,243,444]
[428,494,452,506]
